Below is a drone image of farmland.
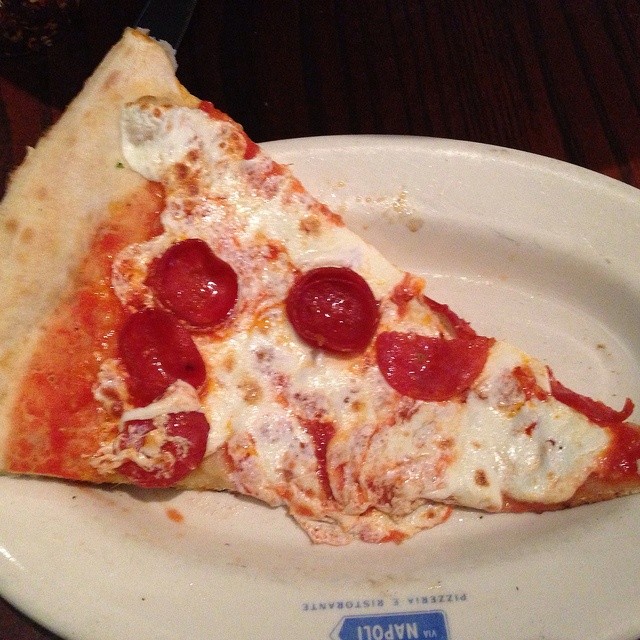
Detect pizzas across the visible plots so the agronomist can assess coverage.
[0,26,640,547]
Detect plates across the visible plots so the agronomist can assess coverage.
[0,132,639,636]
